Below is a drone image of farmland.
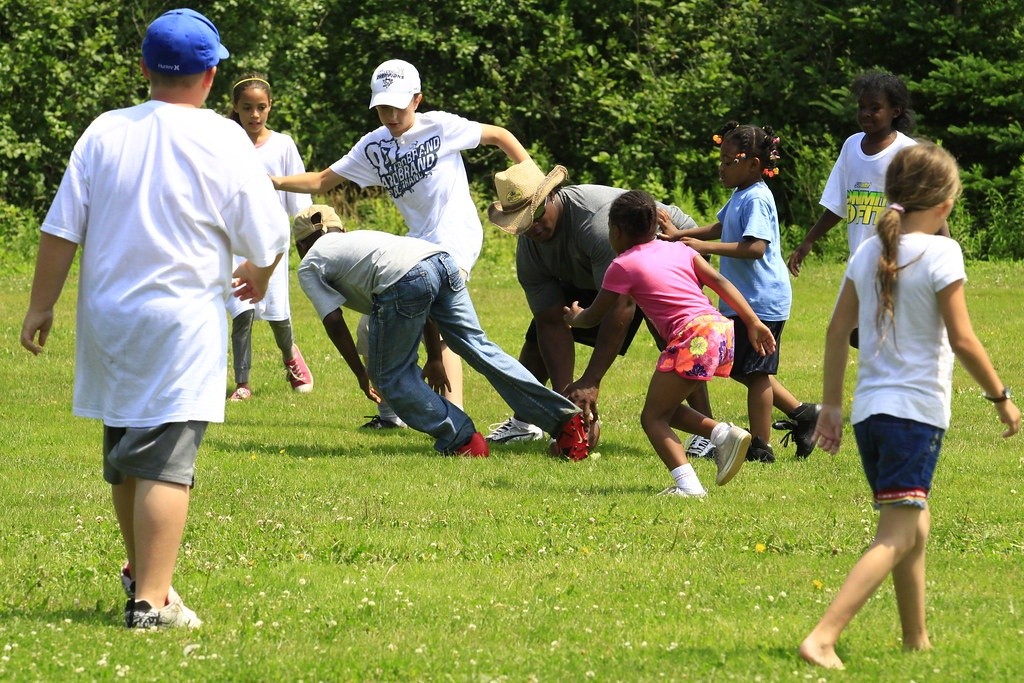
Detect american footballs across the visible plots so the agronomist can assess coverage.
[549,410,600,458]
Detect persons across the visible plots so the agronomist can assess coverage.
[786,68,952,350]
[293,204,589,463]
[20,8,291,633]
[798,140,1022,668]
[483,157,712,444]
[655,120,822,463]
[230,71,314,401]
[267,60,539,429]
[562,189,776,499]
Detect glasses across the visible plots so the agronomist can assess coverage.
[532,205,545,221]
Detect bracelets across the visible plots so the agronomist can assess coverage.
[984,388,1011,403]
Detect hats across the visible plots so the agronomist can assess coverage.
[143,8,230,70]
[487,159,568,234]
[369,60,421,108]
[294,205,345,259]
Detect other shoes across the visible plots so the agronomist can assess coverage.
[360,415,402,429]
[442,431,489,457]
[556,411,588,462]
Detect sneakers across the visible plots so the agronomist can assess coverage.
[484,417,545,442]
[685,432,716,461]
[126,589,203,627]
[741,427,774,462]
[773,401,824,458]
[228,385,251,400]
[121,562,182,607]
[285,342,314,393]
[712,421,751,486]
[658,486,708,498]
